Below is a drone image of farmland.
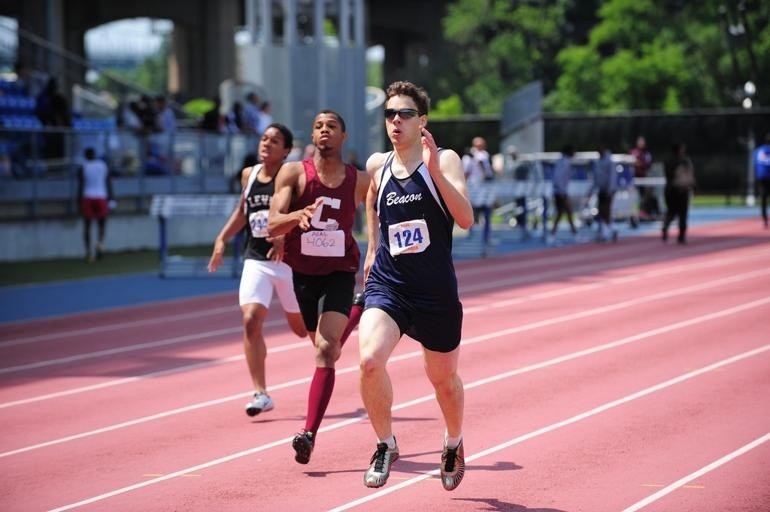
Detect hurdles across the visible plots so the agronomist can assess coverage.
[147,191,248,282]
[445,166,659,266]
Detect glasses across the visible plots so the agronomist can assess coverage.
[383,108,420,119]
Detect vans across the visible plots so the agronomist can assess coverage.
[486,151,671,225]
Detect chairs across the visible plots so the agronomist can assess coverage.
[1,77,248,196]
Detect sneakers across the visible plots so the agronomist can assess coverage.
[292,432,313,465]
[440,436,465,491]
[364,435,399,488]
[468,221,619,245]
[245,394,274,416]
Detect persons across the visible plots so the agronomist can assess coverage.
[34,88,273,176]
[75,146,117,263]
[750,133,770,230]
[358,79,476,492]
[463,133,699,245]
[266,109,378,467]
[205,121,308,417]
